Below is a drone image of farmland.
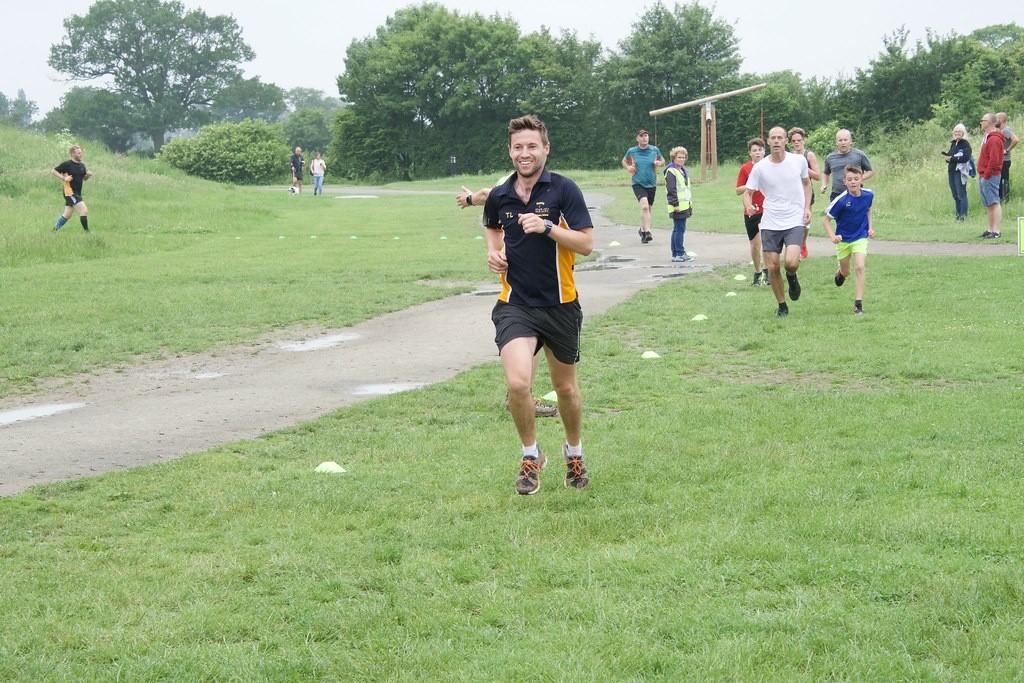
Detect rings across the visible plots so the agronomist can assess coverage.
[748,209,750,210]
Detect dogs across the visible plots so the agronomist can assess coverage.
[288,186,299,197]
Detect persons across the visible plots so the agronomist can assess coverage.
[622,130,665,243]
[821,129,874,202]
[310,152,326,196]
[743,126,813,316]
[482,115,593,495]
[663,147,696,262]
[977,113,1004,240]
[943,123,971,220]
[824,165,875,315]
[735,138,771,287]
[290,147,305,193]
[996,112,1019,204]
[52,145,92,232]
[456,175,557,417]
[788,127,819,259]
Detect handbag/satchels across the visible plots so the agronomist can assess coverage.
[310,160,314,176]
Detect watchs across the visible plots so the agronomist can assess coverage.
[543,220,552,235]
[467,195,473,205]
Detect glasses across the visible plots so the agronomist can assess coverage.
[792,138,803,143]
[978,119,988,122]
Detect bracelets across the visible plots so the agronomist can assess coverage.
[63,177,64,180]
[1006,150,1009,153]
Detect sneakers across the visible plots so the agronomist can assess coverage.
[786,272,802,300]
[853,306,865,315]
[774,308,789,318]
[835,270,846,286]
[763,268,771,284]
[752,271,763,287]
[671,253,695,262]
[560,443,589,489]
[515,445,548,494]
[644,232,652,241]
[505,394,558,417]
[638,229,648,243]
[800,244,808,258]
[980,231,1002,238]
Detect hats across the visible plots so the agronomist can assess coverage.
[638,129,648,136]
